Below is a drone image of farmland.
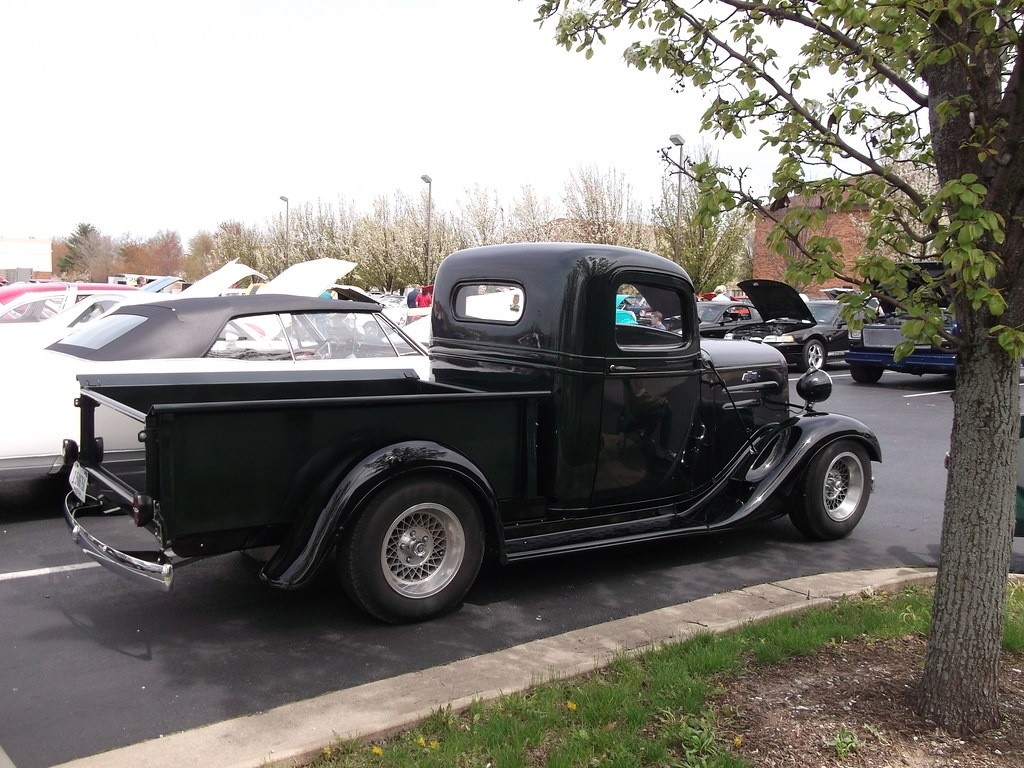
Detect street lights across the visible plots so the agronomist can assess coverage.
[422,175,431,286]
[669,133,685,265]
[279,194,288,270]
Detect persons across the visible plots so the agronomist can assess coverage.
[652,311,666,329]
[712,285,731,301]
[136,276,145,287]
[407,284,432,308]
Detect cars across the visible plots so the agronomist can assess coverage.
[1,257,846,513]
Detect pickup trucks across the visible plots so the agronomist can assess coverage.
[62,243,882,626]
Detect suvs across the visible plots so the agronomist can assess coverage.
[842,260,962,385]
